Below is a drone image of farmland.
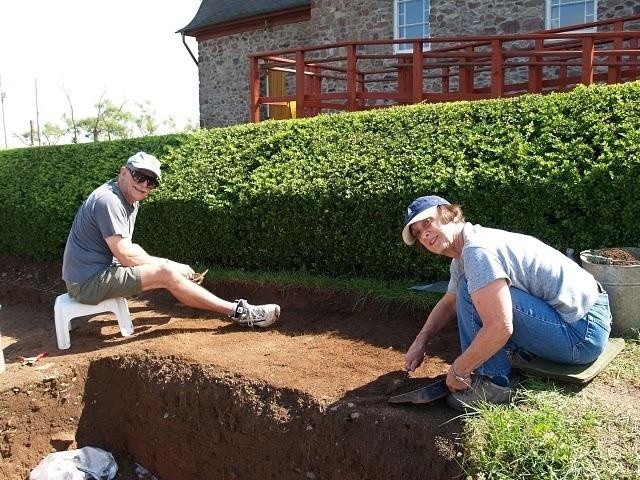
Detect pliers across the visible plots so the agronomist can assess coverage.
[16,351,49,365]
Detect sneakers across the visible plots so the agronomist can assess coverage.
[447,377,512,411]
[229,299,281,328]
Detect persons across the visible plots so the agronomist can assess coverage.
[59,150,282,331]
[384,194,614,413]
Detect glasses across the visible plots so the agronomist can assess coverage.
[126,167,159,189]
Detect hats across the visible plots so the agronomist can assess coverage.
[401,195,452,246]
[126,151,162,181]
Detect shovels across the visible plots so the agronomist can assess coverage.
[386,368,411,395]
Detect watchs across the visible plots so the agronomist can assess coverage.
[450,364,473,381]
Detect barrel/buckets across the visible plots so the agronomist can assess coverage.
[580,247,640,331]
